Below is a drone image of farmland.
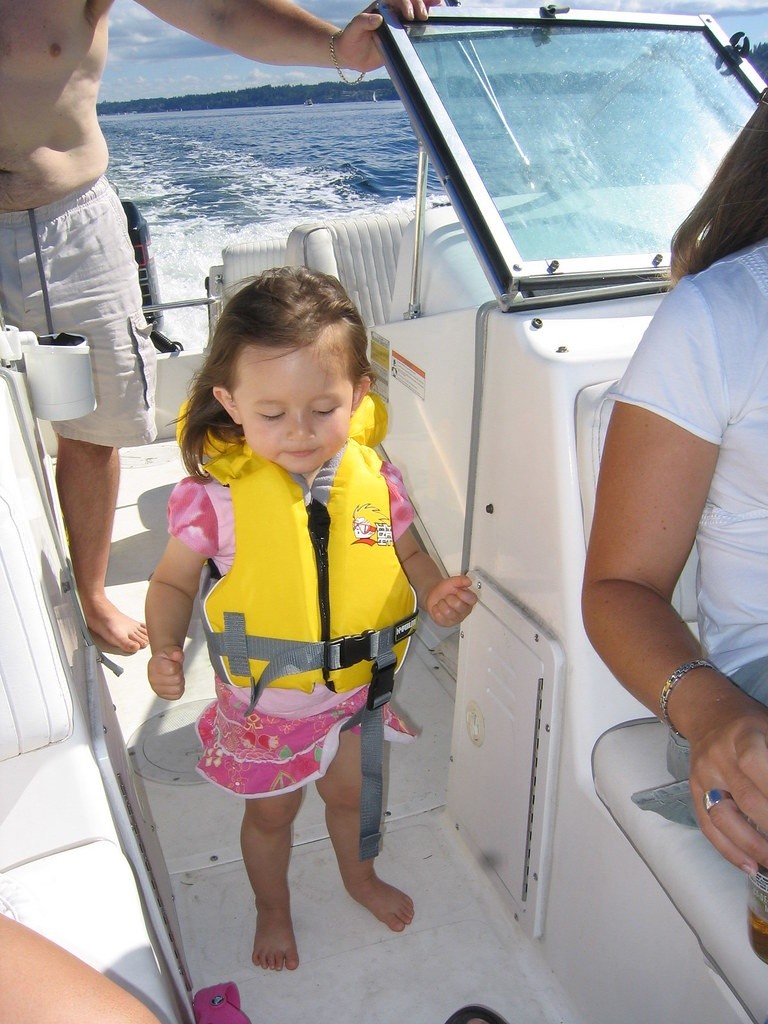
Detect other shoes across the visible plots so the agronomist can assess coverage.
[194,983,251,1024]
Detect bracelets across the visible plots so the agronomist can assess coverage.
[330,30,366,85]
[660,659,722,737]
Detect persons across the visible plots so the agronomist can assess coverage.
[144,265,478,970]
[1,0,442,662]
[582,79,768,879]
[0,912,509,1023]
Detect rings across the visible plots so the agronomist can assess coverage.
[701,788,733,814]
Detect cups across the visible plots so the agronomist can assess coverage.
[747,814,768,965]
[22,332,96,421]
